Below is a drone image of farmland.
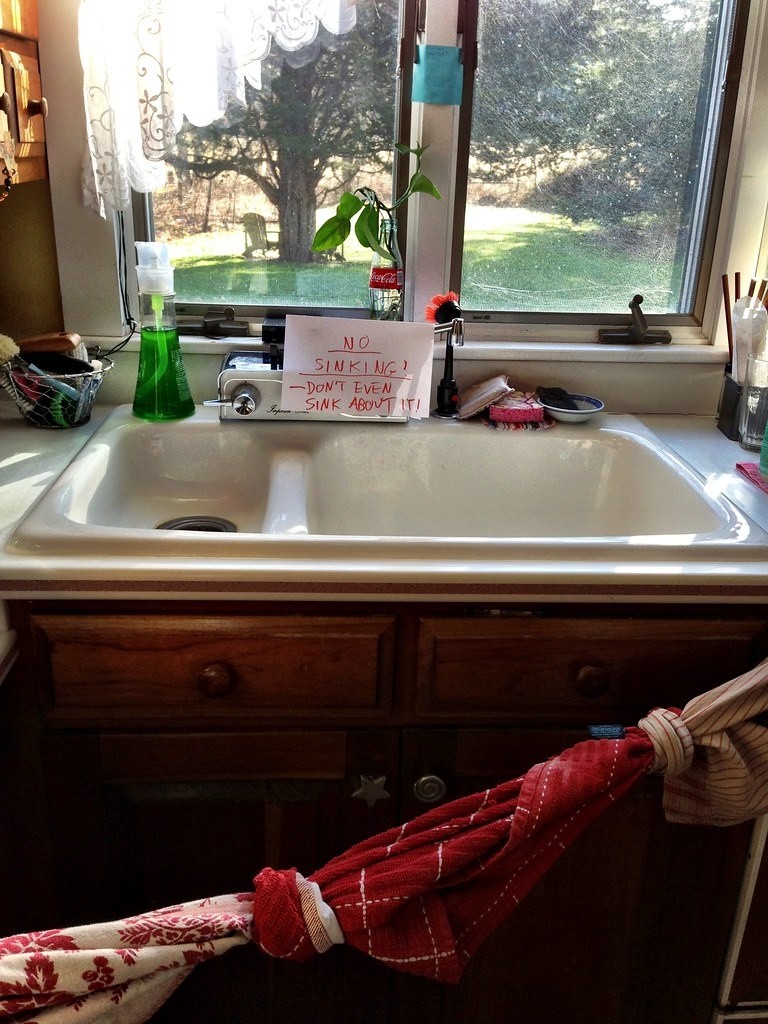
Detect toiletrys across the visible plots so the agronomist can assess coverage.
[132,239,198,423]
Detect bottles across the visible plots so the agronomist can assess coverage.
[369,217,403,322]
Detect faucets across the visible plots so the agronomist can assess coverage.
[434,315,472,355]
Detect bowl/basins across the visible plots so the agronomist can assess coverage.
[537,392,604,423]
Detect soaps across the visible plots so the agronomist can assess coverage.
[534,383,582,412]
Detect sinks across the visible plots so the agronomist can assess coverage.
[287,403,768,566]
[12,392,284,562]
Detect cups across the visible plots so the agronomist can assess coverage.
[713,363,744,443]
[737,352,768,455]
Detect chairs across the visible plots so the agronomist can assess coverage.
[243,212,281,258]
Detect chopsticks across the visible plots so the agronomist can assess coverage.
[720,272,768,378]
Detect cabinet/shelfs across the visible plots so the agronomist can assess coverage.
[387,618,762,1021]
[32,612,400,1022]
[0,0,48,187]
